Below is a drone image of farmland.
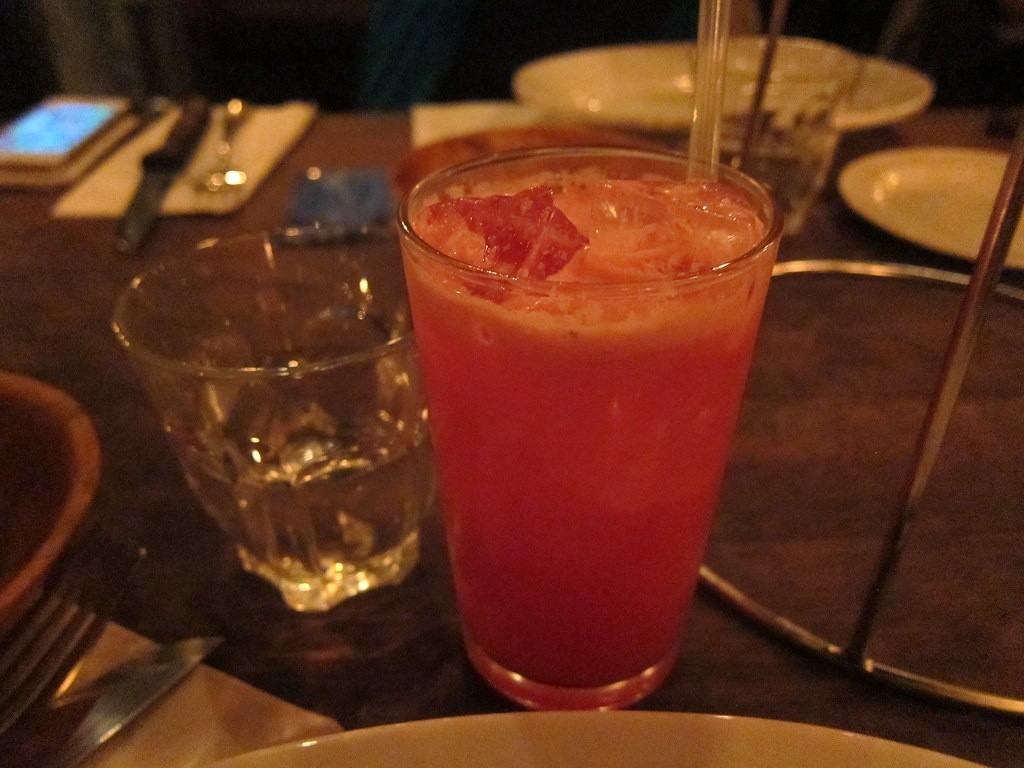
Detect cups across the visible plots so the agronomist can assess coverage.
[688,38,863,233]
[109,219,439,612]
[393,142,785,708]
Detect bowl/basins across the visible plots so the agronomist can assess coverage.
[0,368,101,631]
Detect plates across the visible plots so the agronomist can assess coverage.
[509,34,934,132]
[836,142,1024,269]
[190,709,979,768]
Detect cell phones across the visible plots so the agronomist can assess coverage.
[270,164,397,246]
[1,90,133,170]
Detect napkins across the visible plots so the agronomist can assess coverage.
[48,103,319,218]
[0,592,345,768]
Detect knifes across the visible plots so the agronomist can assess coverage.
[0,635,219,768]
[114,95,212,255]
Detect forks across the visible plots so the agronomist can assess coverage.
[0,580,100,742]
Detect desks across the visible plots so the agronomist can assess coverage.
[0,104,1024,768]
